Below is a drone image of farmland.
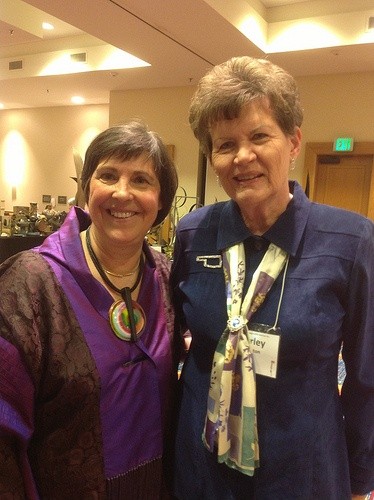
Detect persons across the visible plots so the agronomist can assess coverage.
[168,57,374,500]
[0,119,189,500]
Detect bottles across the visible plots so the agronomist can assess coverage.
[2,212,11,236]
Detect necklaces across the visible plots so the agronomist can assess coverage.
[85,224,147,340]
